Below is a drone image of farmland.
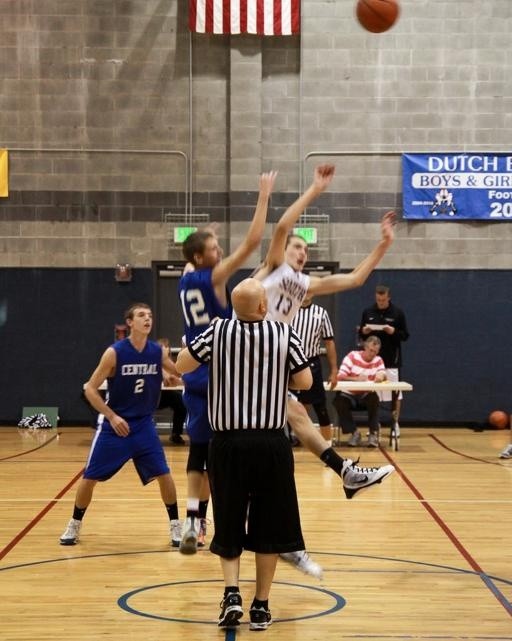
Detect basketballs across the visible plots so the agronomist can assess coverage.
[357,0,399,33]
[491,411,506,428]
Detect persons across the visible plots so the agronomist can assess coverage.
[158,159,411,579]
[59,300,183,548]
[497,440,512,461]
[176,277,314,631]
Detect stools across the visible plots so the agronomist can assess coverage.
[338,412,381,448]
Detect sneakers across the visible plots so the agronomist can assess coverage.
[341,422,400,499]
[59,518,82,545]
[499,444,512,458]
[169,435,321,630]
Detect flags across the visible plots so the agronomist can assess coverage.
[186,0,302,39]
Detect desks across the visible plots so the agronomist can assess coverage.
[319,381,414,448]
[82,379,186,437]
